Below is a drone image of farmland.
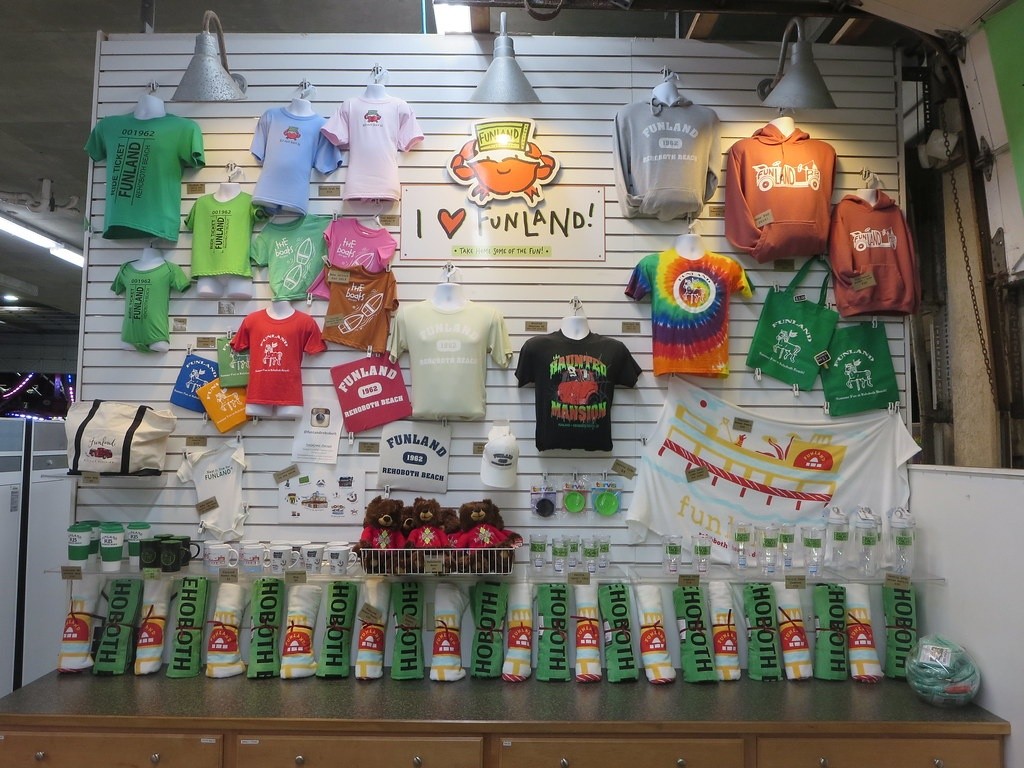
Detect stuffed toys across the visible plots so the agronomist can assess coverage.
[350,495,523,573]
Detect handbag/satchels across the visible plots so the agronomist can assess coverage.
[65,400,177,477]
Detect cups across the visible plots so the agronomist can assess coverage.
[98,521,125,572]
[126,522,151,566]
[691,534,712,574]
[139,533,358,575]
[551,533,610,575]
[527,535,548,568]
[660,534,683,574]
[730,523,825,578]
[67,524,91,571]
[80,520,101,567]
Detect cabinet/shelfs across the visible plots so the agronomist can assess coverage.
[0,672,1010,768]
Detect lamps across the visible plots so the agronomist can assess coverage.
[757,17,837,114]
[168,7,247,106]
[465,12,544,102]
[0,210,84,267]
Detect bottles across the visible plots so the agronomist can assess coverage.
[826,506,849,572]
[855,507,914,579]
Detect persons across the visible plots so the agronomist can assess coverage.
[625,234,756,378]
[247,98,344,216]
[82,95,205,244]
[612,81,722,222]
[230,299,326,420]
[183,182,268,300]
[828,189,921,319]
[722,117,836,262]
[319,84,424,201]
[513,314,643,451]
[111,247,191,353]
[386,283,513,421]
